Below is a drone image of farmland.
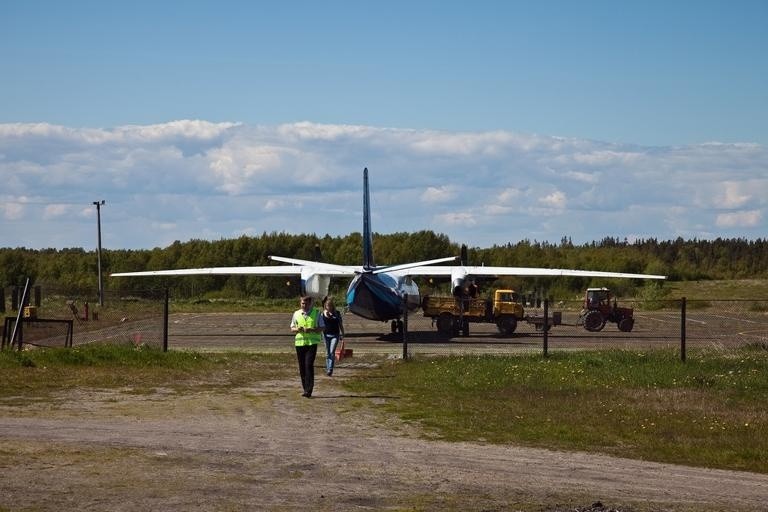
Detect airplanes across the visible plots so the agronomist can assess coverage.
[108,169,669,337]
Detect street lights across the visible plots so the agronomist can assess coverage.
[93,200,107,306]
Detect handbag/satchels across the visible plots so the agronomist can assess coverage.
[335,340,345,362]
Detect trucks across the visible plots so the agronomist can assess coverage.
[419,282,521,335]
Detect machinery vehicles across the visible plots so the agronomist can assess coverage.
[582,287,635,333]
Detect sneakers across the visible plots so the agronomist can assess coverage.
[301,391,312,398]
[327,372,332,375]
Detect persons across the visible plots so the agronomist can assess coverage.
[453,279,479,313]
[318,298,344,376]
[290,296,326,399]
